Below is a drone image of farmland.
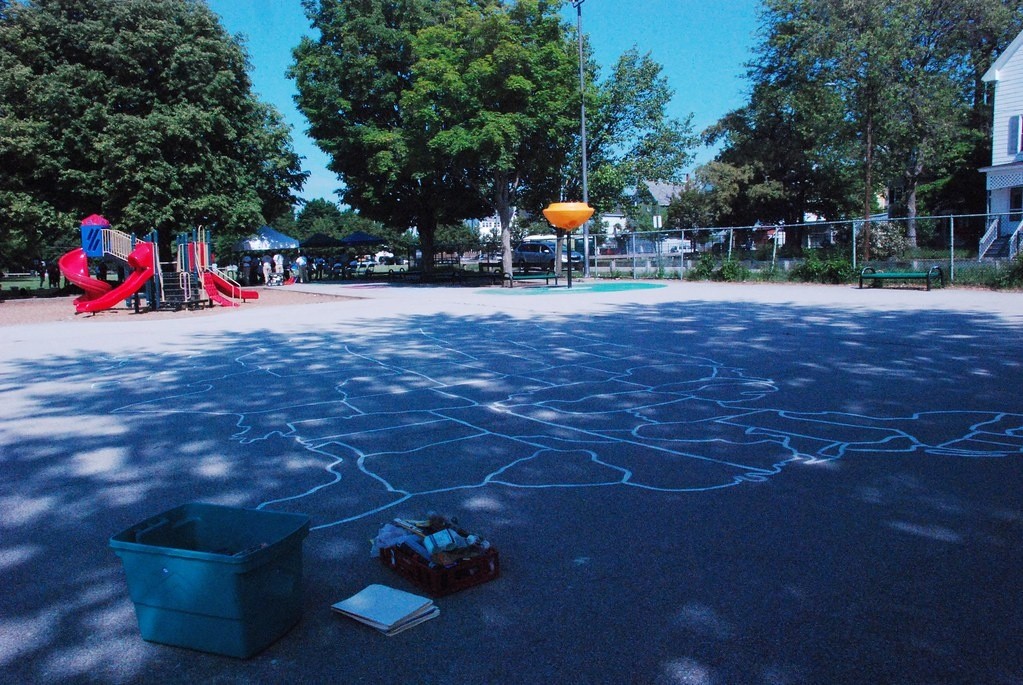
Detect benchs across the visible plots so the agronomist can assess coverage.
[501,270,557,288]
[365,268,503,286]
[859,265,945,291]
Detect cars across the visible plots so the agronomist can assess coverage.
[353,254,409,274]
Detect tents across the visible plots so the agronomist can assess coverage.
[340,229,387,246]
[300,232,348,251]
[232,225,300,261]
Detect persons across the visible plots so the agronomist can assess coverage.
[95,262,100,279]
[244,248,359,285]
[98,261,107,280]
[47,263,61,287]
[38,261,46,287]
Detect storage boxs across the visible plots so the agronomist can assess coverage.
[107,501,313,658]
[379,538,501,598]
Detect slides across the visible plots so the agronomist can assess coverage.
[58,248,111,304]
[212,272,260,299]
[77,267,155,313]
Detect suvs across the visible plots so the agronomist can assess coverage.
[513,241,583,273]
[671,245,699,255]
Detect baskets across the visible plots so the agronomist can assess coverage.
[378,541,501,597]
